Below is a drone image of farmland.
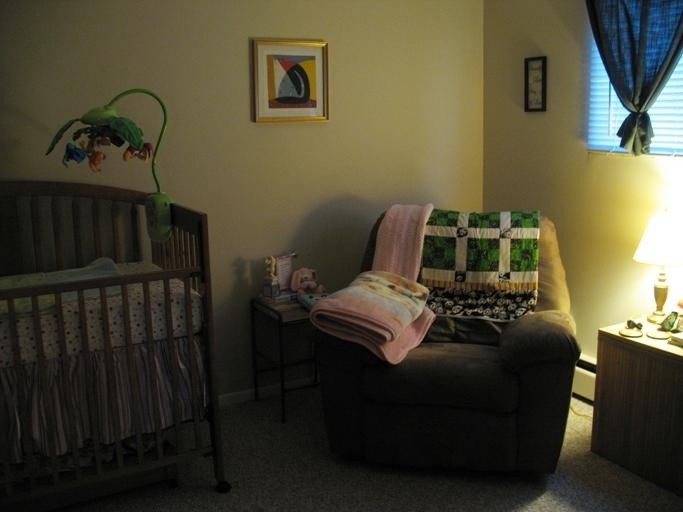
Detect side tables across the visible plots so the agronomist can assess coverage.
[251,296,322,421]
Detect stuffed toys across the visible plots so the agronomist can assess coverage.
[291,268,327,295]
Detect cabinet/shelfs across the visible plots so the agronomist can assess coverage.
[591,316,683,493]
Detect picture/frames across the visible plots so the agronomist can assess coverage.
[524,55,546,110]
[253,38,330,121]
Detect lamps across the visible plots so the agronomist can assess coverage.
[632,211,682,322]
[45,87,173,244]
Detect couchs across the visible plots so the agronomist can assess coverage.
[315,211,581,483]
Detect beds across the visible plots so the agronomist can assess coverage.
[2,180,227,512]
